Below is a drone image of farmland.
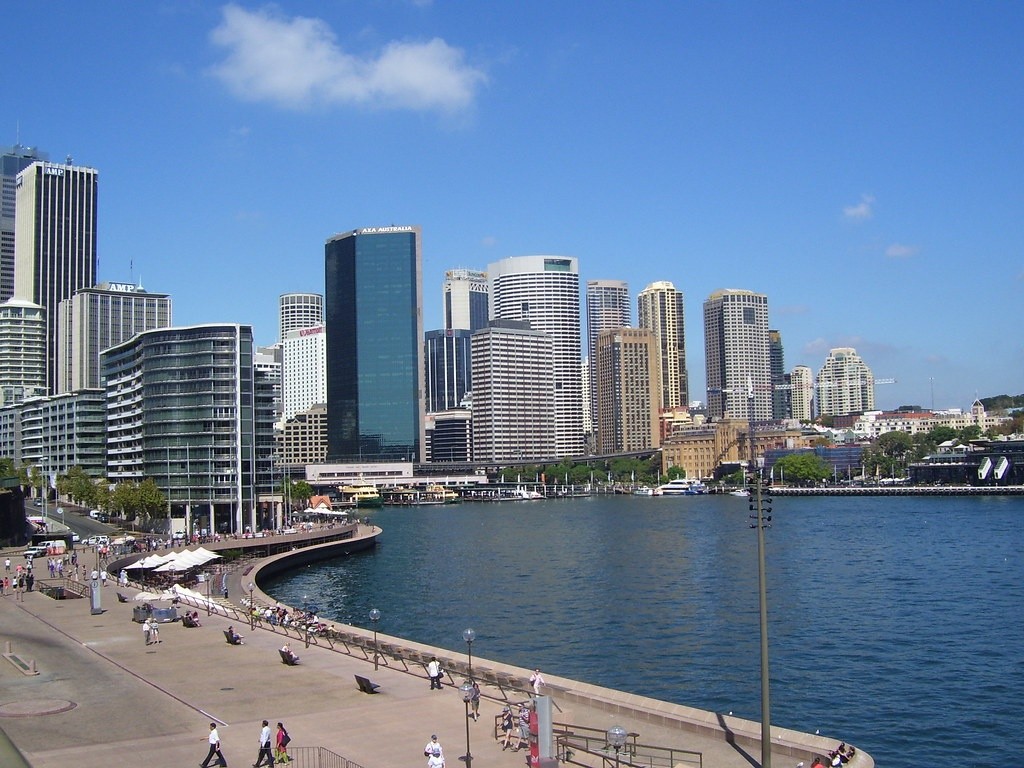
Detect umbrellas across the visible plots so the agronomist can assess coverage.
[121,545,224,581]
[304,507,349,524]
[133,590,180,608]
[168,584,214,602]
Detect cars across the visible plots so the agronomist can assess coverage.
[81,535,135,555]
[90,510,109,522]
[173,531,184,539]
[34,500,44,507]
[73,532,80,540]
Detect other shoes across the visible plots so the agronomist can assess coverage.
[220,765,226,767]
[273,760,278,764]
[507,745,512,747]
[503,746,507,750]
[512,747,518,752]
[199,764,205,768]
[284,762,289,766]
[268,765,274,768]
[252,764,259,768]
[525,746,530,751]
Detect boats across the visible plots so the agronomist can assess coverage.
[634,480,707,496]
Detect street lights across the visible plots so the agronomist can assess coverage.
[301,593,313,648]
[247,581,256,630]
[139,558,148,591]
[169,564,179,598]
[462,627,475,683]
[203,572,214,617]
[360,444,368,461]
[369,609,382,670]
[40,457,48,521]
[608,725,627,768]
[458,683,476,768]
[745,462,777,768]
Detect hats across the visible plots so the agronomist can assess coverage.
[433,749,441,755]
[432,735,437,739]
[502,707,509,712]
[517,702,524,707]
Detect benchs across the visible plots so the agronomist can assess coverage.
[223,630,244,645]
[116,593,128,602]
[278,649,300,666]
[322,628,522,695]
[180,614,201,628]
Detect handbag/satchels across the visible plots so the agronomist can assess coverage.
[281,735,291,747]
[531,682,534,686]
[438,672,443,678]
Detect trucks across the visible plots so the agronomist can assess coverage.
[23,540,65,558]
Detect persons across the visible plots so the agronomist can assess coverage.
[811,742,855,768]
[469,683,481,721]
[252,720,290,768]
[137,591,200,645]
[126,516,369,553]
[265,600,339,637]
[282,643,299,661]
[530,668,545,697]
[498,703,530,752]
[47,539,128,588]
[199,722,227,768]
[425,735,445,768]
[229,625,244,645]
[429,657,443,690]
[24,525,43,541]
[0,553,34,602]
